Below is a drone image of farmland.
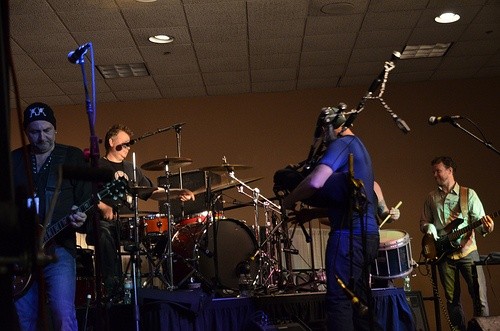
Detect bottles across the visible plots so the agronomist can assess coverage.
[238,273,249,298]
[245,273,254,297]
[403,273,412,291]
[123,274,133,304]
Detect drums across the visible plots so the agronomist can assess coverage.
[129,213,174,236]
[171,210,223,237]
[163,217,262,295]
[371,228,416,279]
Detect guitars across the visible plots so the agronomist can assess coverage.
[422,210,500,266]
[0,172,132,305]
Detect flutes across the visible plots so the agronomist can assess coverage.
[272,50,403,214]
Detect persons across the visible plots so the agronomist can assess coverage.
[85,123,196,303]
[419,156,494,331]
[283,107,380,331]
[10,101,93,331]
[320,179,400,288]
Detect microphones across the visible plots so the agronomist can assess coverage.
[115,143,126,150]
[239,264,250,274]
[68,42,91,64]
[197,242,213,258]
[428,115,465,125]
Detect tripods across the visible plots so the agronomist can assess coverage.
[225,166,329,297]
[102,162,223,305]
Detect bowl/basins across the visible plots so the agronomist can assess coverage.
[188,283,201,289]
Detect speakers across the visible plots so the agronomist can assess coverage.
[466,316,500,331]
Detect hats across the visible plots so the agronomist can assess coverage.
[23,102,57,130]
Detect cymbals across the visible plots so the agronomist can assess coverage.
[210,175,265,193]
[203,163,253,172]
[141,156,192,172]
